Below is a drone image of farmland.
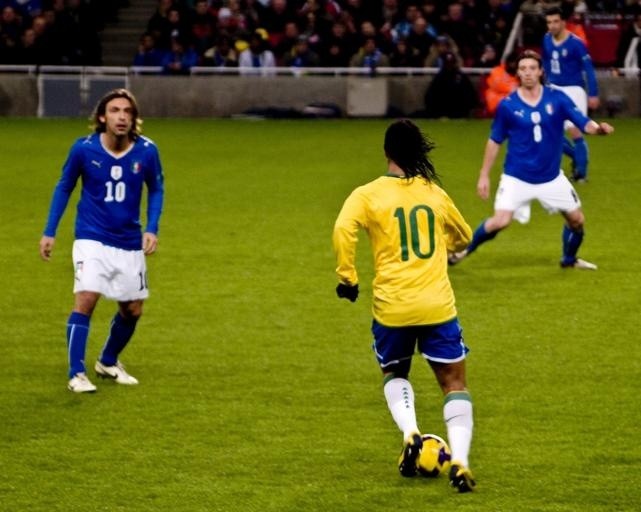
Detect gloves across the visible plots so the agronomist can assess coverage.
[336,283,359,302]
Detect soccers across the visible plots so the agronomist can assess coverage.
[414,433,451,477]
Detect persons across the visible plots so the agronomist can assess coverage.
[332,120,475,494]
[39,88,164,393]
[447,51,614,270]
[1,2,640,76]
[540,7,601,183]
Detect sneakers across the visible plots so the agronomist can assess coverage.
[448,460,476,492]
[94,357,139,385]
[448,248,469,265]
[399,432,422,477]
[561,258,598,270]
[67,372,97,393]
